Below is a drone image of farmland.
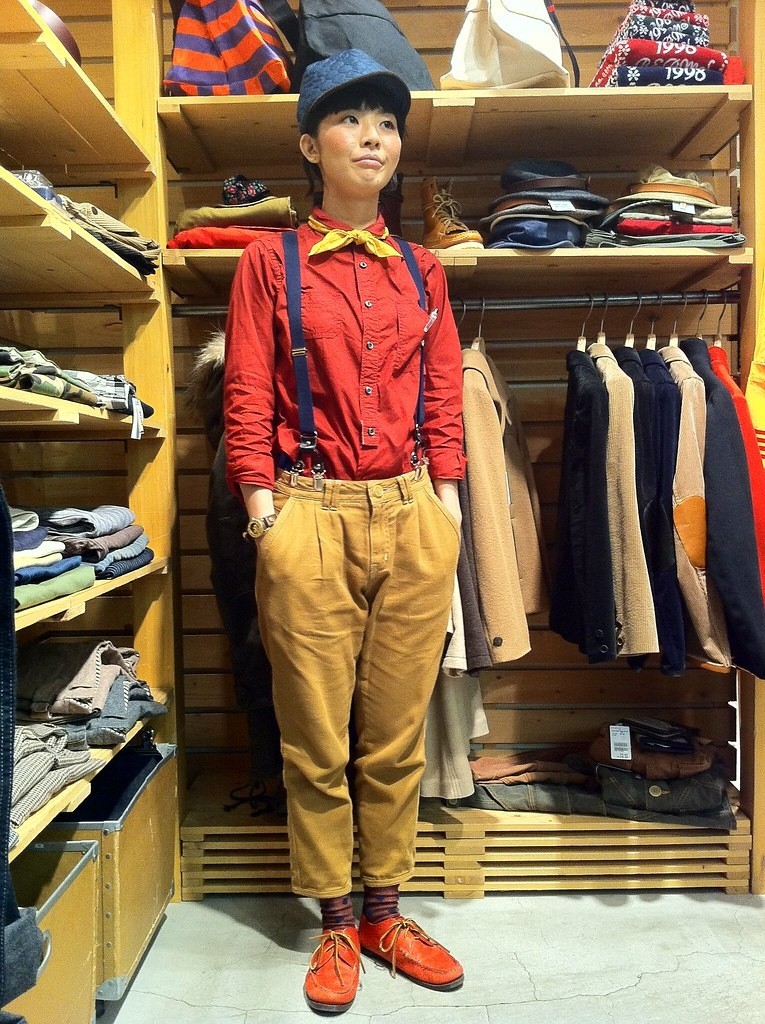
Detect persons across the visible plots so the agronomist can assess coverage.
[225,48,467,1015]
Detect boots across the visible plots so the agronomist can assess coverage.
[420,175,484,249]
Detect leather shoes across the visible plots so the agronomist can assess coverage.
[303,925,360,1013]
[358,915,464,991]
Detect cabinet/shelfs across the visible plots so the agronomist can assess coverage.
[0,0,765,1024]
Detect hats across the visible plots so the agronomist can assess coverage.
[296,49,411,138]
[479,157,747,249]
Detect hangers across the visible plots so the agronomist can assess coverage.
[645,291,663,350]
[596,295,610,345]
[667,291,688,346]
[471,297,488,358]
[454,297,467,333]
[713,288,727,346]
[622,292,642,348]
[575,297,594,353]
[693,289,709,339]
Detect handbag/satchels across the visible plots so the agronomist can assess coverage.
[440,0,581,88]
[163,0,295,96]
[260,0,437,90]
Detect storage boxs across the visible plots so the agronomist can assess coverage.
[37,742,177,1001]
[0,839,98,1024]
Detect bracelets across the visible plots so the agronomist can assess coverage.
[243,515,276,538]
